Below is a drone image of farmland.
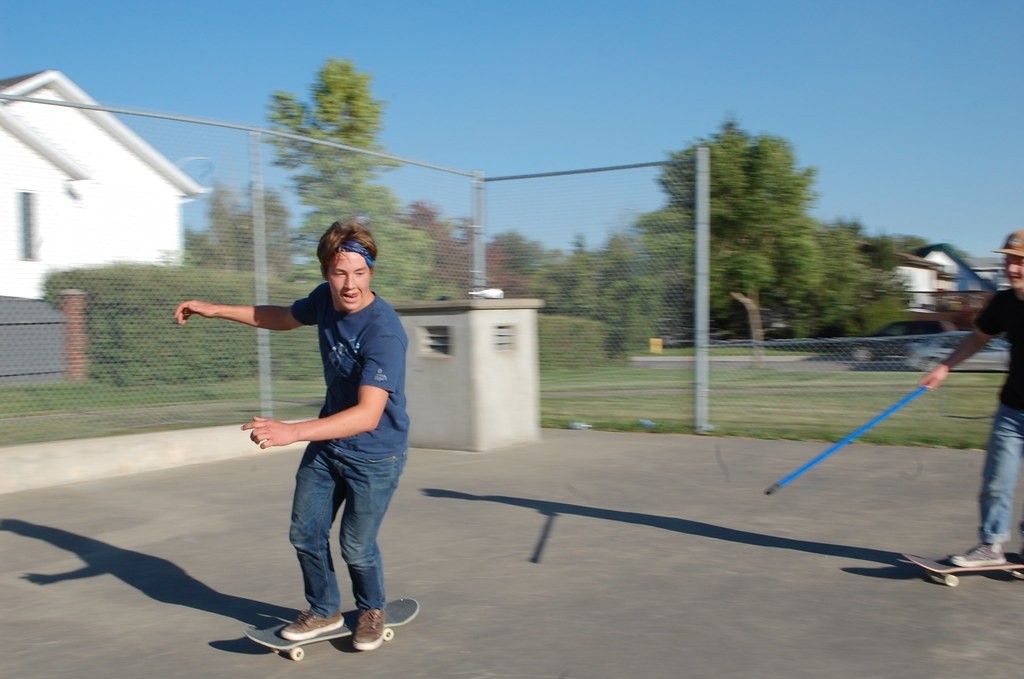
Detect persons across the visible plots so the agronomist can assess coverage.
[173,217,410,650]
[914,229,1024,569]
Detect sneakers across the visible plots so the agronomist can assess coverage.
[352,606,386,652]
[949,543,1007,567]
[280,609,345,641]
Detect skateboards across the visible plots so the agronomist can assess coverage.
[241,598,421,663]
[901,545,1024,588]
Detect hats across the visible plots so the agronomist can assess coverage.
[991,229,1024,257]
